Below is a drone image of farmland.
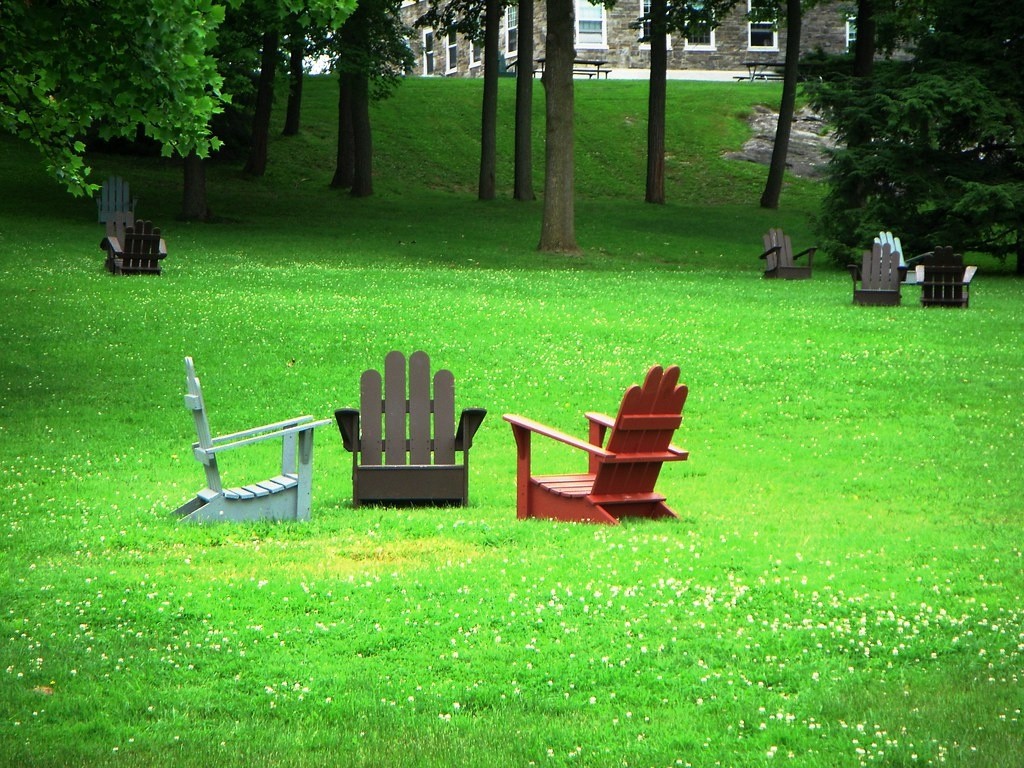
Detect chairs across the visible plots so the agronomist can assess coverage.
[94,175,168,276]
[502,363,689,525]
[170,354,334,523]
[761,228,818,281]
[846,230,977,308]
[334,349,488,509]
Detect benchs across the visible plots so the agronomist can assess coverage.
[532,69,612,79]
[733,74,784,82]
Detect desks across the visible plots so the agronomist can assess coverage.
[742,61,786,82]
[538,58,608,78]
